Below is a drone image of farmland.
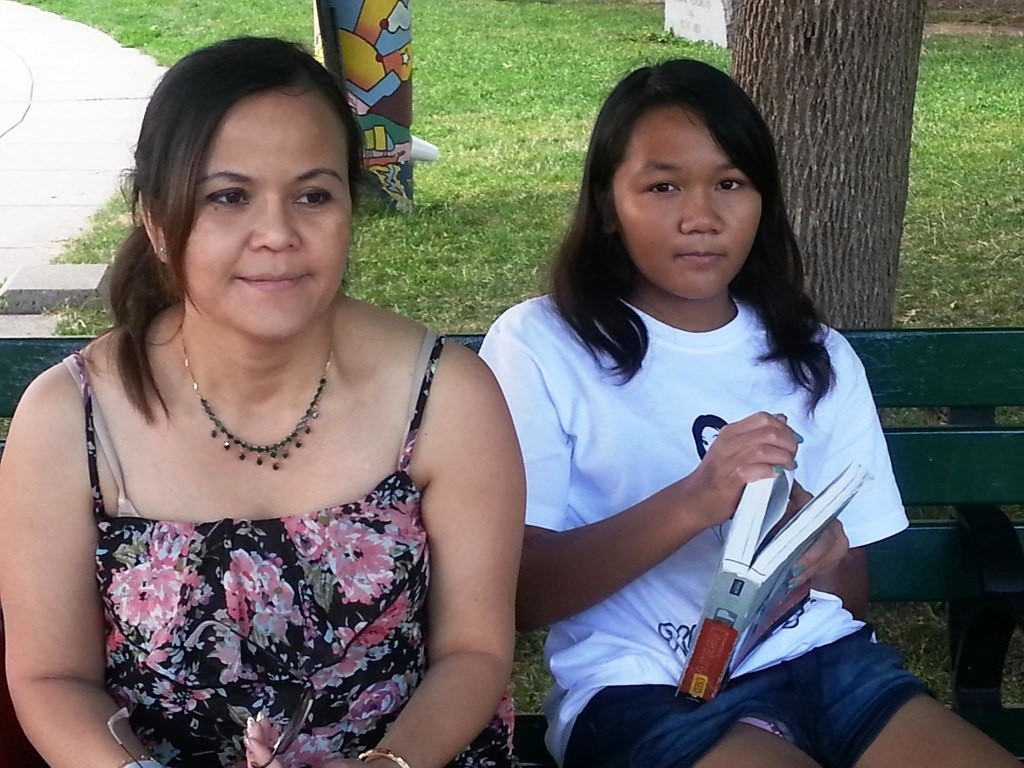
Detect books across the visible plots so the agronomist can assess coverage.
[676,414,868,705]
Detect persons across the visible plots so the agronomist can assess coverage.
[478,59,1024,768]
[2,37,528,768]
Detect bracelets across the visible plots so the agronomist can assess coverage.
[356,749,410,768]
[122,756,163,768]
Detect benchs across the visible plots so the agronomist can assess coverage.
[0,328,1024,766]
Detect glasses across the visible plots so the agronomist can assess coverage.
[108,620,316,768]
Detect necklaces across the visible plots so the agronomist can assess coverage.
[180,339,332,470]
